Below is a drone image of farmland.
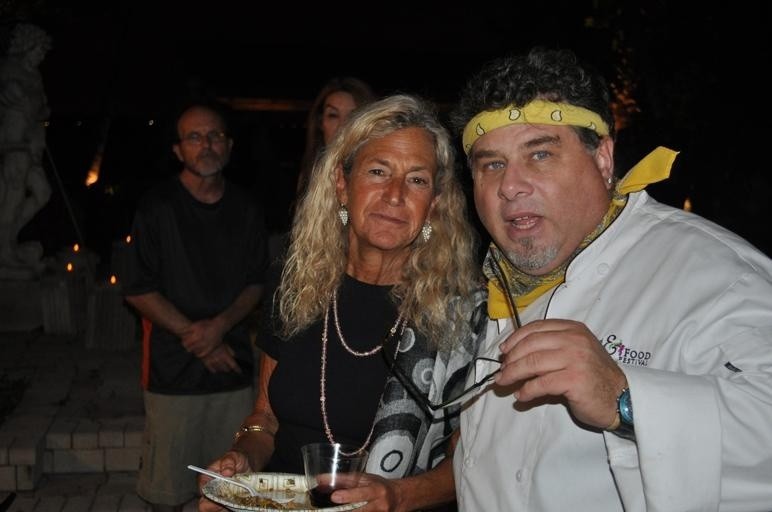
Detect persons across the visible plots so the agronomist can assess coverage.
[287,77,373,210]
[117,104,275,511]
[194,90,489,511]
[448,41,770,512]
[0,21,55,269]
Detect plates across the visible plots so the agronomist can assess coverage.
[201,471,369,512]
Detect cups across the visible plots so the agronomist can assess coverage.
[301,441,368,507]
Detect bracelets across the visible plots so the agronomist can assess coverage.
[230,423,282,448]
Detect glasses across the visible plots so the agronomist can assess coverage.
[179,129,226,146]
[383,248,521,411]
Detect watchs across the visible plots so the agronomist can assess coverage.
[614,385,638,441]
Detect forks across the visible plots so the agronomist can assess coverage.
[188,464,294,505]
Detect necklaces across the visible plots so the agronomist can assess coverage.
[318,298,413,457]
[331,285,410,359]
